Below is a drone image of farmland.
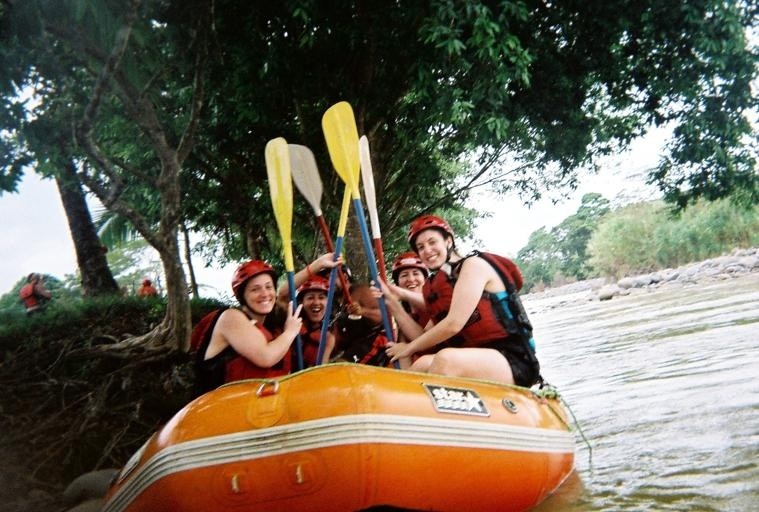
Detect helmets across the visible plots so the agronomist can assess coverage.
[32,273,41,280]
[407,213,455,254]
[322,263,353,283]
[295,274,331,301]
[231,260,277,306]
[143,280,151,286]
[391,251,429,280]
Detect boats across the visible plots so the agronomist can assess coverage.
[90,360,580,512]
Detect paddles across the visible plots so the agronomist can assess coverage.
[265,101,402,369]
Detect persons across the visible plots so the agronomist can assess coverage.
[139,280,156,296]
[19,274,51,315]
